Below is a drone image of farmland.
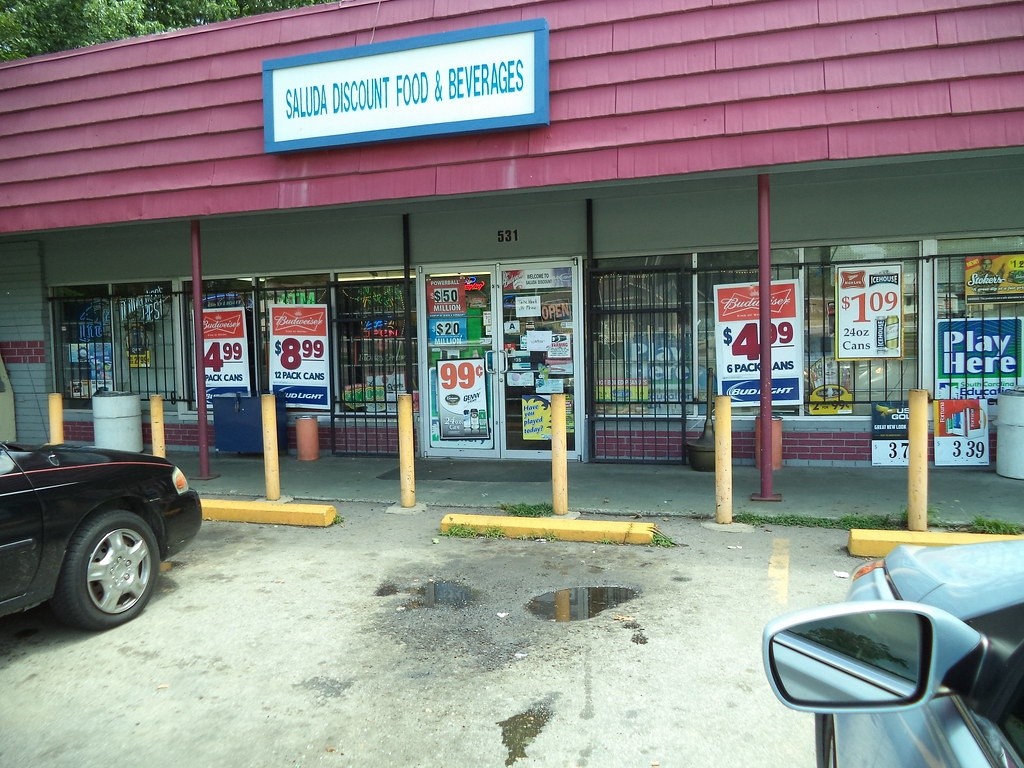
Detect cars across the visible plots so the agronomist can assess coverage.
[0,441,201,631]
[763,538,1024,768]
